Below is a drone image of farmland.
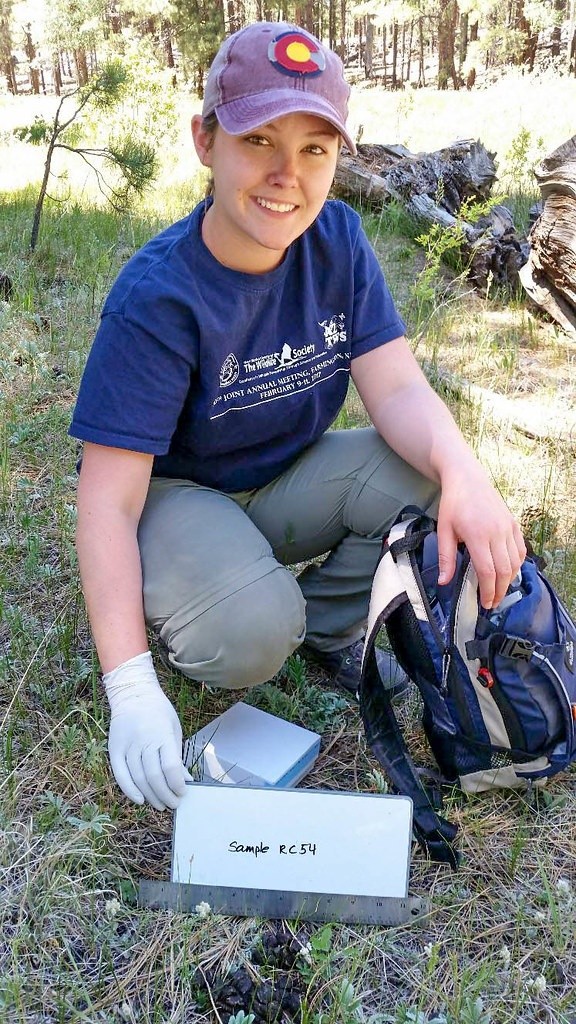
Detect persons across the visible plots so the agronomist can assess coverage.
[69,22,526,812]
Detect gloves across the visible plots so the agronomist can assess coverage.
[102,650,193,813]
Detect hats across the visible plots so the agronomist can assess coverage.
[201,22,358,156]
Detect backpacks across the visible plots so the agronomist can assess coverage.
[359,505,576,873]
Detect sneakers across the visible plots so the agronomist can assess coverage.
[300,636,409,705]
[158,638,203,688]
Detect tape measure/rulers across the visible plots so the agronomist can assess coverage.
[138,879,431,929]
